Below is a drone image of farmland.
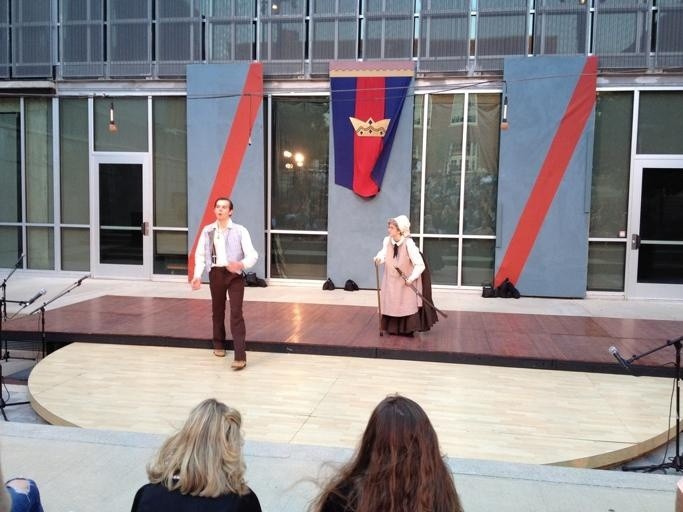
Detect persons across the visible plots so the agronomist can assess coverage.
[314,392,463,511]
[372,213,425,336]
[188,197,260,372]
[127,396,262,512]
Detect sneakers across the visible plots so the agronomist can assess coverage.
[232,360,246,368]
[214,350,225,356]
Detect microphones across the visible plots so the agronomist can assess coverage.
[76,273,92,283]
[109,102,117,134]
[15,253,25,266]
[25,288,46,306]
[610,345,631,372]
[499,96,508,131]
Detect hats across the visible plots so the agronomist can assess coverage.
[387,214,410,235]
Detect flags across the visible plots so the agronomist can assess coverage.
[328,76,413,201]
